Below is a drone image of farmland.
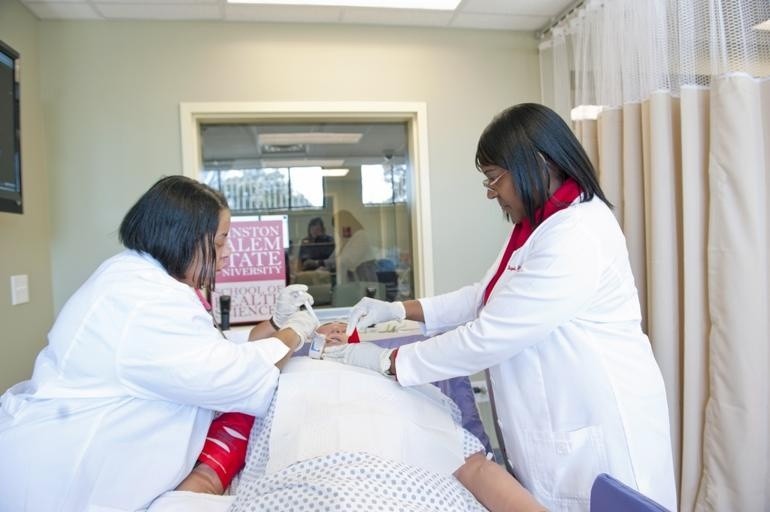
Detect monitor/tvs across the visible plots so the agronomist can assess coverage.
[0,40,21,213]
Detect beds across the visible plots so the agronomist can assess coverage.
[289,334,672,511]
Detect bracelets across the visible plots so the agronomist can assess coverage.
[269,316,280,332]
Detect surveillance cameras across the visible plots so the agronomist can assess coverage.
[382,150,395,161]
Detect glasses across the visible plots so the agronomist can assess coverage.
[481,166,516,195]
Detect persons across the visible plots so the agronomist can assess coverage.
[321,102,678,511]
[174,320,545,511]
[298,216,327,270]
[332,209,379,282]
[0,175,321,511]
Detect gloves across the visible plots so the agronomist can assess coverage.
[277,311,319,352]
[270,283,316,328]
[344,295,409,339]
[321,342,400,376]
